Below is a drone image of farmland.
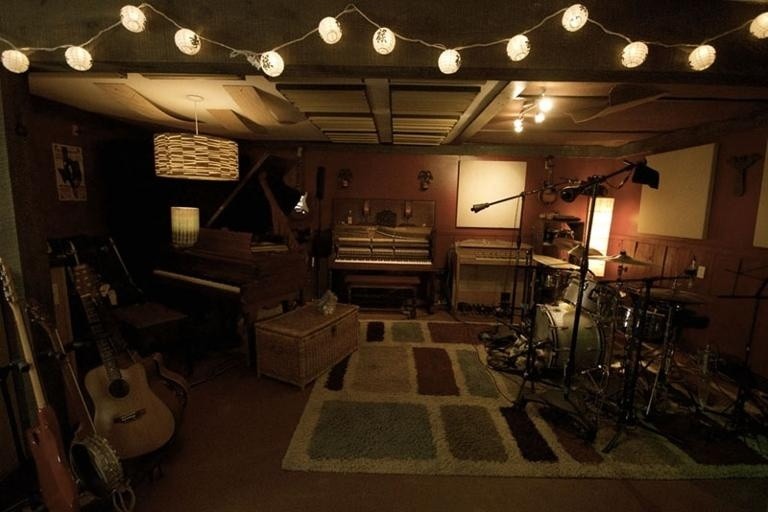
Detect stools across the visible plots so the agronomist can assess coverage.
[115,301,188,364]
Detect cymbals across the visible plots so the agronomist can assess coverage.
[644,288,710,306]
[589,252,652,271]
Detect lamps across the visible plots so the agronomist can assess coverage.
[505,87,553,135]
[148,93,244,183]
[167,204,201,249]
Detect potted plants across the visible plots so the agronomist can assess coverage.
[337,168,355,189]
[416,168,433,190]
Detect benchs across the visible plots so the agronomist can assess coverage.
[341,273,421,319]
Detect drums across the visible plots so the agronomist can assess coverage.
[536,304,601,375]
[532,252,576,300]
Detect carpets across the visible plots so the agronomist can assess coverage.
[283,319,768,478]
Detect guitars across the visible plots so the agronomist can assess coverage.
[82,261,190,419]
[0,256,133,510]
[61,243,176,457]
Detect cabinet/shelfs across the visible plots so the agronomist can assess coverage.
[535,214,585,266]
